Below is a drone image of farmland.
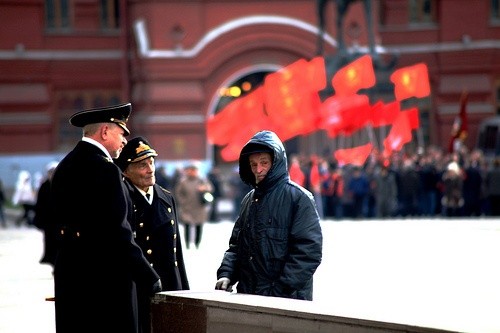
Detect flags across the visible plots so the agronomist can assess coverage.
[204,48,431,197]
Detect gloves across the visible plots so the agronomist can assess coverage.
[143,279,162,297]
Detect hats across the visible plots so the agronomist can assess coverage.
[70,102,132,137]
[241,146,273,156]
[114,137,159,170]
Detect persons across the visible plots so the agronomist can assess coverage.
[215,130,323,301]
[154,162,225,225]
[175,166,213,248]
[112,137,190,333]
[46,102,162,333]
[227,144,500,222]
[0,159,60,230]
